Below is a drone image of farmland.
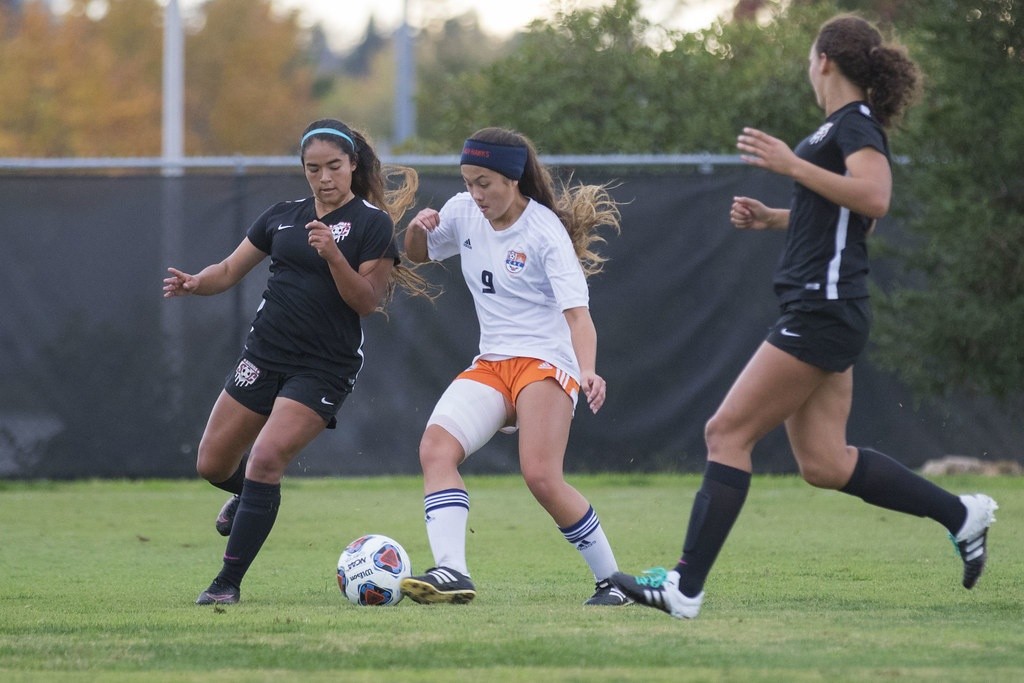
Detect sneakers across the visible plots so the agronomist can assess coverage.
[610,569,709,622]
[215,495,240,537]
[195,575,240,605]
[582,577,635,608]
[400,565,477,607]
[952,490,1000,589]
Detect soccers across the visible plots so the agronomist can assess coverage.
[337,533,413,609]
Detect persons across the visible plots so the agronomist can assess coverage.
[164,118,444,604]
[401,127,635,606]
[611,15,997,621]
